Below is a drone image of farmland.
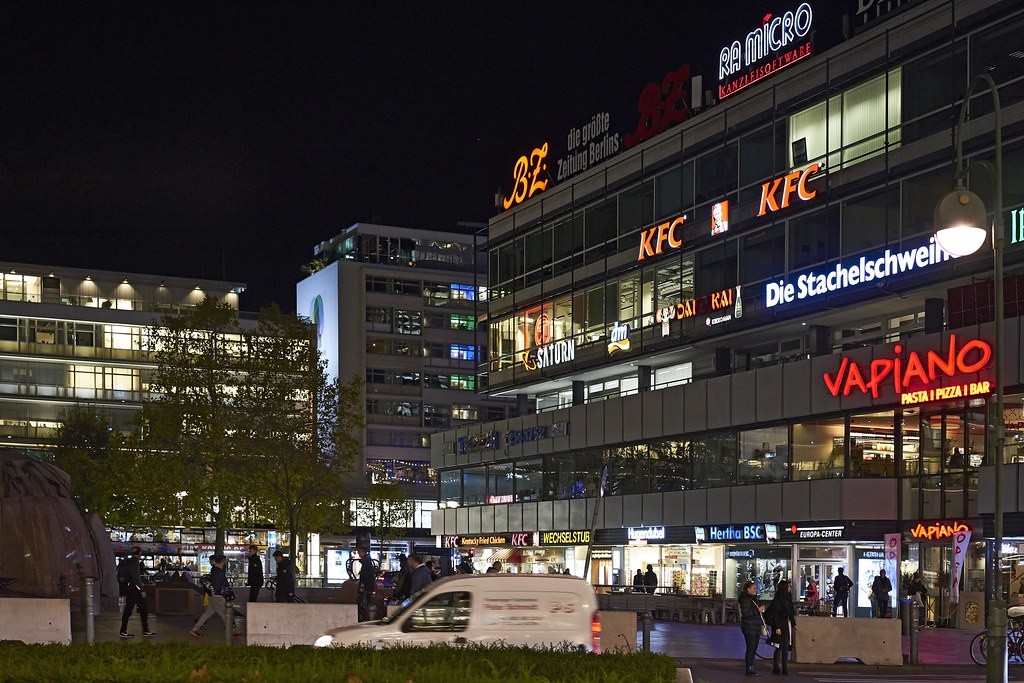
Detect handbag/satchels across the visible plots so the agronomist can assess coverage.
[760,625,769,636]
[915,591,924,607]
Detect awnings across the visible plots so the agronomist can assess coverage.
[486,548,521,563]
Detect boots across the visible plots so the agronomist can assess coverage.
[746,665,760,676]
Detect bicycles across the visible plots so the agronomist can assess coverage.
[756,605,817,660]
[970,619,1024,665]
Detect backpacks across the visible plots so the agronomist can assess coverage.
[116,557,139,598]
[763,597,786,627]
[201,570,221,598]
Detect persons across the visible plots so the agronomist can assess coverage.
[189,554,241,639]
[247,545,264,602]
[116,558,168,585]
[433,599,467,627]
[748,563,756,583]
[869,569,892,618]
[394,554,438,606]
[458,563,473,598]
[972,578,1024,594]
[119,546,159,638]
[833,568,853,617]
[947,446,963,468]
[633,569,645,593]
[907,575,927,627]
[806,576,818,616]
[550,568,572,575]
[272,550,296,603]
[644,565,658,595]
[771,563,783,595]
[766,581,797,675]
[738,581,765,676]
[356,547,375,622]
[486,562,501,574]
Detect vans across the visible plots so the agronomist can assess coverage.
[314,572,602,657]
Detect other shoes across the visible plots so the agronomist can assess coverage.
[120,630,134,638]
[774,669,781,674]
[233,632,241,638]
[782,670,791,676]
[142,629,157,636]
[190,629,203,638]
